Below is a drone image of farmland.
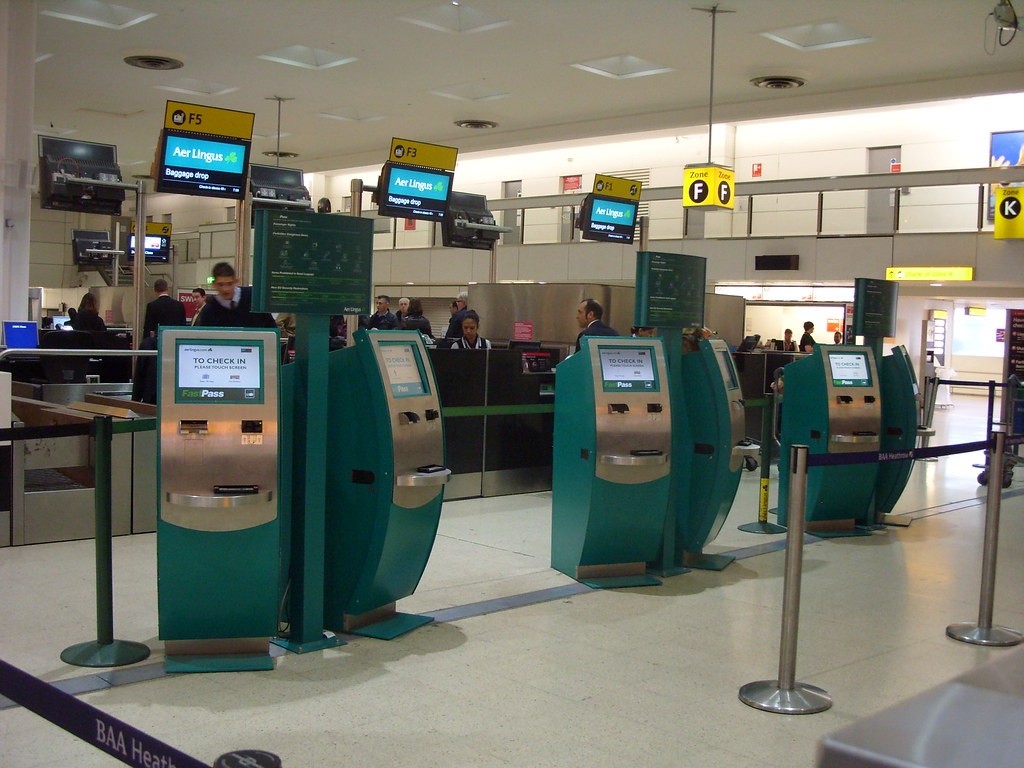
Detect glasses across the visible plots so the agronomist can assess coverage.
[456,301,462,303]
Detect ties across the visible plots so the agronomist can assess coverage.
[230,300,235,310]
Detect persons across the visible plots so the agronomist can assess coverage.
[64,307,77,326]
[630,326,657,337]
[367,295,400,330]
[451,309,491,349]
[71,293,108,330]
[405,298,433,337]
[200,262,277,328]
[800,321,816,352]
[834,331,841,343]
[784,329,795,351]
[396,298,410,320]
[445,291,468,338]
[330,315,344,337]
[448,300,458,315]
[190,288,207,327]
[276,314,296,338]
[574,298,620,354]
[143,279,186,337]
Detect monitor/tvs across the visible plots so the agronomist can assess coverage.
[71,229,113,266]
[175,338,265,405]
[828,351,873,387]
[37,134,125,217]
[598,345,660,393]
[379,340,432,398]
[156,130,252,201]
[128,233,170,262]
[248,164,312,228]
[579,192,639,245]
[441,191,500,250]
[53,315,71,331]
[378,160,454,222]
[716,351,739,390]
